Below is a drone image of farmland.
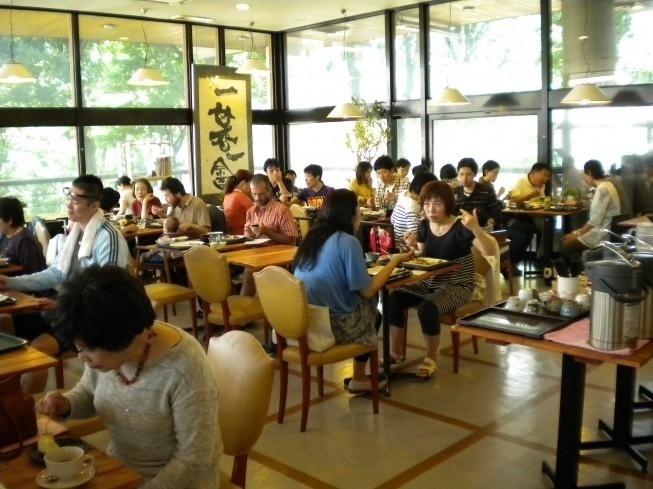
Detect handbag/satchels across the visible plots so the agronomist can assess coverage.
[270,303,336,352]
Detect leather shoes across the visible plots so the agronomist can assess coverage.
[347,377,388,395]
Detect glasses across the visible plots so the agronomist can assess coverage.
[63,186,91,201]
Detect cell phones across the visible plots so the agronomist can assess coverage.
[252,224,259,228]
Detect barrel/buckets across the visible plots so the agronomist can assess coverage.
[585,234,652,350]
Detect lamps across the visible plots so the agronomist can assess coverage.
[127,7,170,86]
[428,1,470,106]
[0,0,37,84]
[325,9,365,120]
[561,1,615,105]
[234,22,270,74]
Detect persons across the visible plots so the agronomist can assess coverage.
[34,262,223,488]
[480,152,623,276]
[222,152,498,395]
[1,175,209,393]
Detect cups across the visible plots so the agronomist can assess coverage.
[42,446,94,480]
[106,207,150,233]
[499,259,591,320]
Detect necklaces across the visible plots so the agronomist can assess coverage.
[111,328,154,384]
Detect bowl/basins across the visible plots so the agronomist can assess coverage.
[506,191,582,213]
[358,205,387,223]
[0,258,9,264]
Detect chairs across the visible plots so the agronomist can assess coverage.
[0,187,653,489]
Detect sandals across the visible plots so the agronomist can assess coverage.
[378,352,405,366]
[416,357,439,378]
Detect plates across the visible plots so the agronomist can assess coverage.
[24,440,90,465]
[153,230,253,248]
[149,217,167,228]
[34,469,96,489]
[360,249,449,283]
[0,264,9,269]
[0,295,17,305]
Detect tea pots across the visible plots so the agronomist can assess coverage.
[625,221,652,252]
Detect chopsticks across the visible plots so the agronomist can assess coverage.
[393,236,417,262]
